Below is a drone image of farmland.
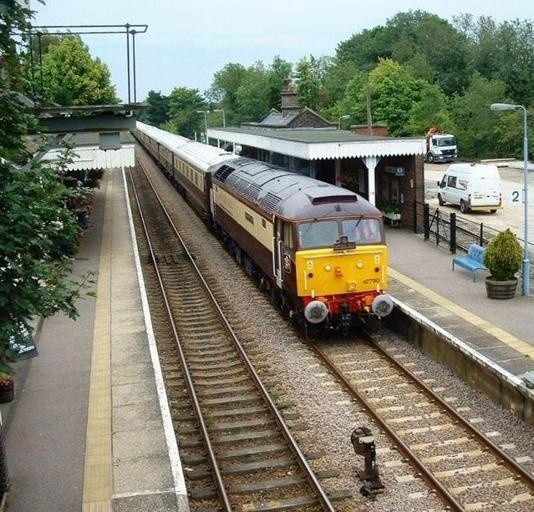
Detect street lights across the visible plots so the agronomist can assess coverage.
[488,102,532,297]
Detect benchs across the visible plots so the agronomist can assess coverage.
[452,244,493,282]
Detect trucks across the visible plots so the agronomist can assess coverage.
[421,127,459,165]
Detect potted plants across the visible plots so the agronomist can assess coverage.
[484,229,525,299]
[375,201,402,227]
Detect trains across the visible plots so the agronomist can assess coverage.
[127,118,395,344]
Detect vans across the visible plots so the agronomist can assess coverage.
[436,162,504,215]
